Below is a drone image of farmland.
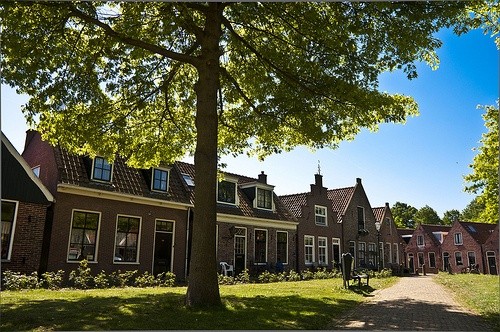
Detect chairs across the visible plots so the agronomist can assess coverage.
[340,252,369,289]
